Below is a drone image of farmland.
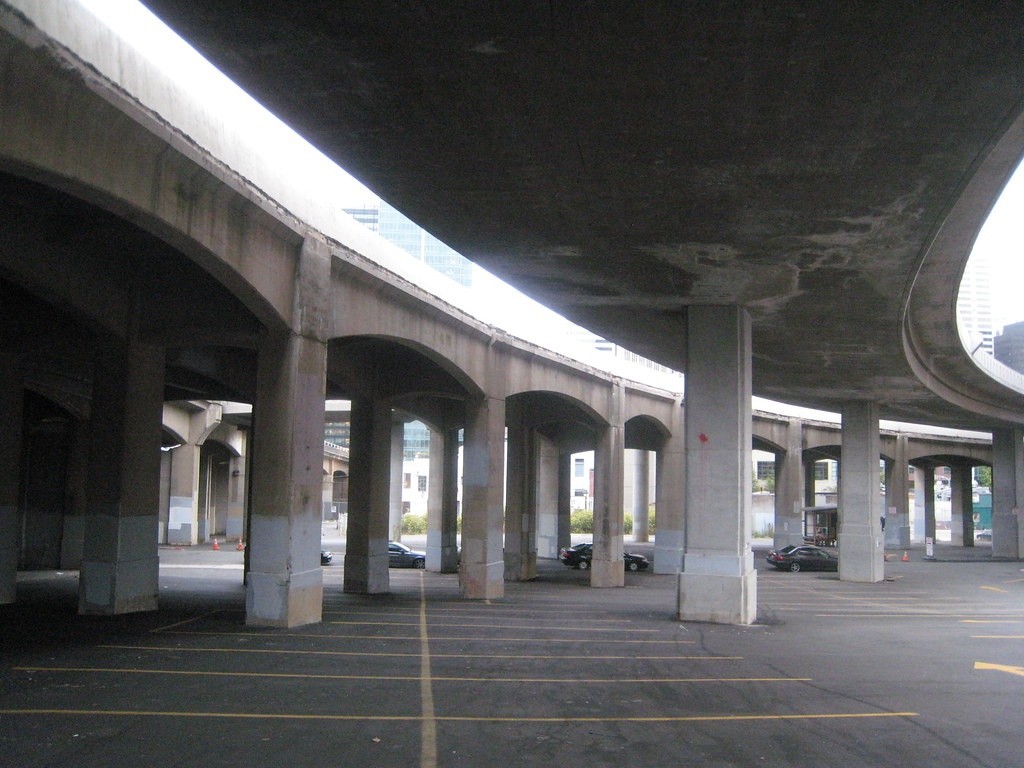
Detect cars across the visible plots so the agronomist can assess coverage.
[388,541,426,569]
[766,544,838,572]
[558,543,648,572]
[977,530,992,542]
[321,550,332,564]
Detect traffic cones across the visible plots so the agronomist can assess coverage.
[902,550,909,562]
[236,539,244,550]
[212,538,220,550]
